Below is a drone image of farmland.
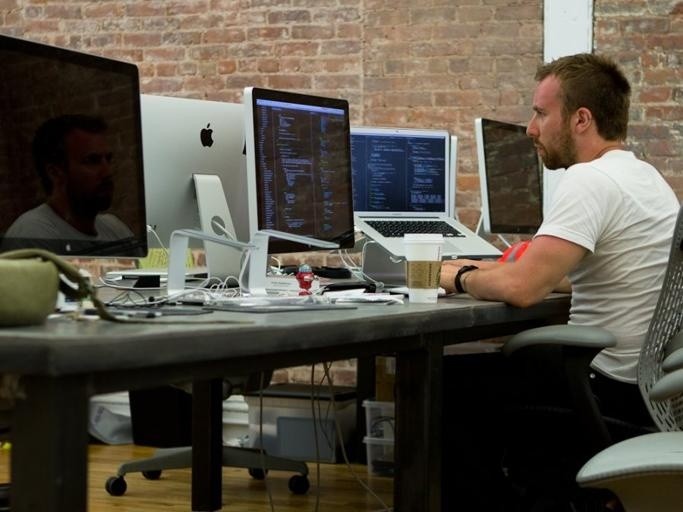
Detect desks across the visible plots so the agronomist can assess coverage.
[0,292,572,512]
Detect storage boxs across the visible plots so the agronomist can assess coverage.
[244,382,357,458]
[362,398,395,438]
[363,436,395,475]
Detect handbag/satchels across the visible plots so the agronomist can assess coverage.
[0,249,91,326]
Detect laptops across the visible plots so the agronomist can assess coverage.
[348,126,505,264]
[103,268,209,283]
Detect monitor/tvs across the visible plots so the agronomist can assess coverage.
[238,86,355,293]
[141,94,249,290]
[0,34,149,261]
[473,117,542,248]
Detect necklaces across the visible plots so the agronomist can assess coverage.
[590,144,624,161]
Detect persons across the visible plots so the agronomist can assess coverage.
[424,55,683,510]
[5,111,140,245]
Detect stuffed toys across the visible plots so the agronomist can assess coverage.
[295,263,317,296]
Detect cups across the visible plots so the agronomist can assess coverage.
[402,231,446,304]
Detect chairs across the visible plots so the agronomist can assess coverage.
[576,348,683,512]
[106,248,311,497]
[506,206,682,432]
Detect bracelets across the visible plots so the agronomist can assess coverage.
[454,264,479,294]
